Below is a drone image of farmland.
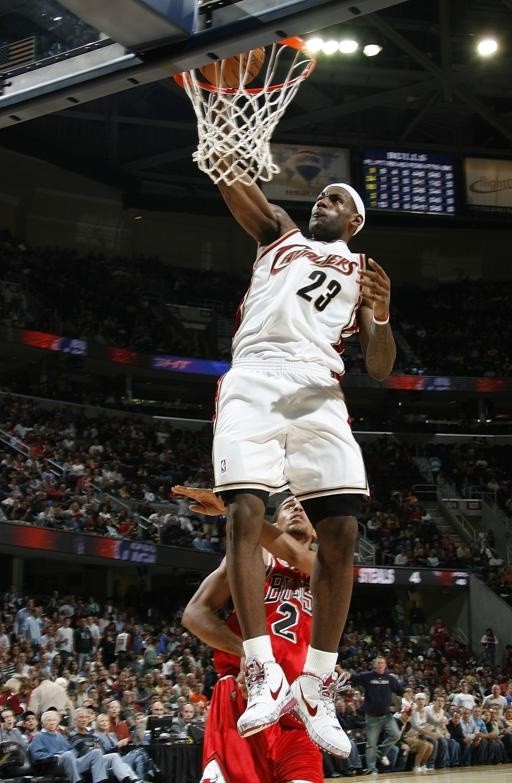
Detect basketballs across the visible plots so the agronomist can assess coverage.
[200,45,268,90]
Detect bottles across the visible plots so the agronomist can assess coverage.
[143,730,151,745]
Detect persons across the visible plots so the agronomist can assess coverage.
[3,583,218,781]
[343,280,510,376]
[1,365,227,555]
[202,92,397,761]
[323,595,511,778]
[2,231,253,356]
[350,413,510,597]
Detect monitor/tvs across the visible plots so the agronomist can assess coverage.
[146,715,173,731]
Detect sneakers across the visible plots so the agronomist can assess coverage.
[381,756,390,766]
[237,655,297,738]
[288,671,352,759]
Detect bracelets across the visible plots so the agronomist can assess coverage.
[369,310,392,326]
